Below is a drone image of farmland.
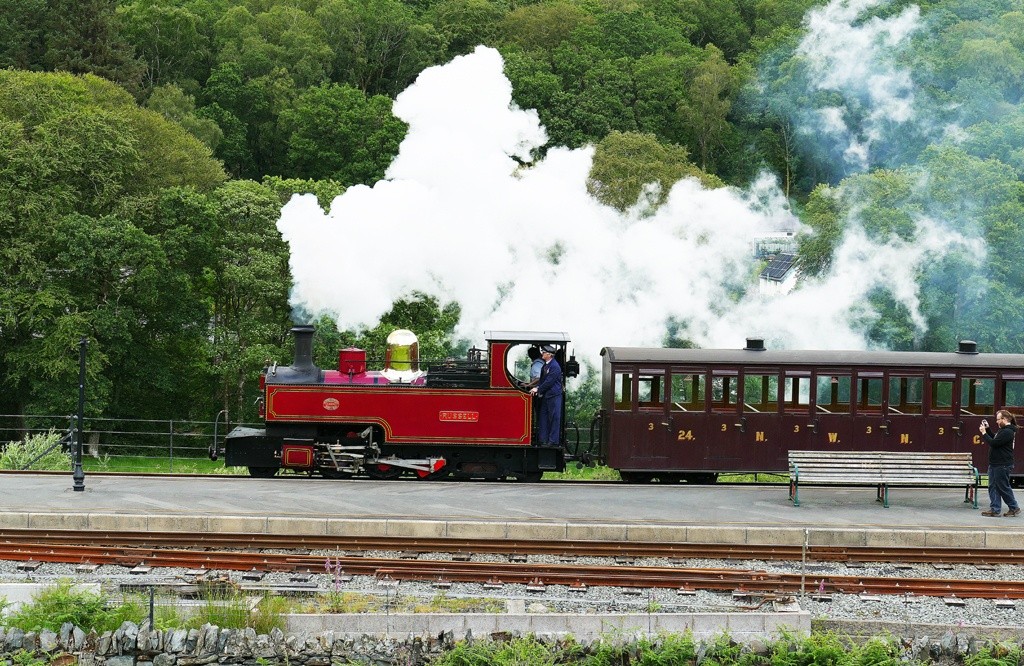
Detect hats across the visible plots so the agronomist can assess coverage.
[541,346,557,353]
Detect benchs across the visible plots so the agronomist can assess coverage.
[787,449,979,510]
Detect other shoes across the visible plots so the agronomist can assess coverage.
[540,443,559,447]
[982,510,1001,517]
[1004,508,1022,518]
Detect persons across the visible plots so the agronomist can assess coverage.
[527,345,562,447]
[979,410,1021,517]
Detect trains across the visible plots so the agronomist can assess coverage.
[224,325,1020,487]
